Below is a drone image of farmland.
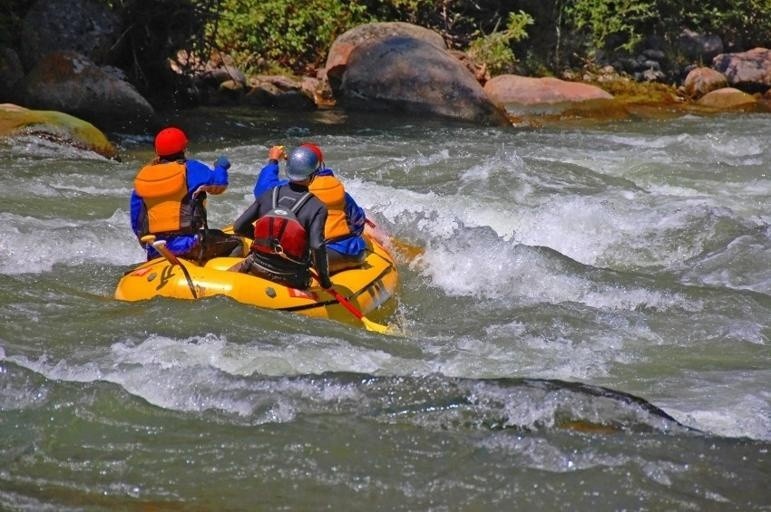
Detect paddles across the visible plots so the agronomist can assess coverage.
[309,271,389,333]
[364,217,425,262]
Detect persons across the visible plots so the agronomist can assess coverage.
[130,128,244,265]
[233,148,332,290]
[255,144,366,274]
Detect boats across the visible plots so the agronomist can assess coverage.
[112,220,399,327]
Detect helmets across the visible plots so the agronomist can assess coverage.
[154,127,188,156]
[286,143,323,181]
